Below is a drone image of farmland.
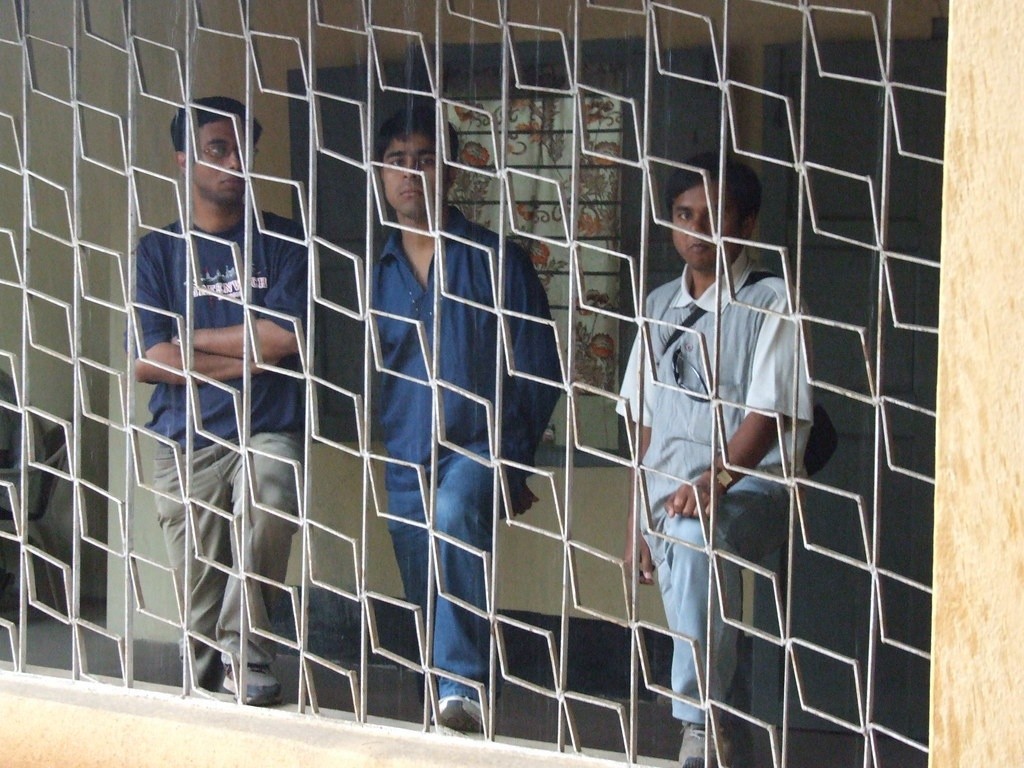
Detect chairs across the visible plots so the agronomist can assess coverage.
[0,369,73,614]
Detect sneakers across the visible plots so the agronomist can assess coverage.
[222,663,281,696]
[430,695,483,733]
[679,720,715,768]
[709,720,733,758]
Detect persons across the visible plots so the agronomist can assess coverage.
[615,153,812,768]
[373,109,562,730]
[124,96,312,703]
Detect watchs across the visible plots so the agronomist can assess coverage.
[705,463,735,495]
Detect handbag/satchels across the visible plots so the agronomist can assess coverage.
[803,404,837,476]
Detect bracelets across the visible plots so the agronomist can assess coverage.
[176,334,181,344]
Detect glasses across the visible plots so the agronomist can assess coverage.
[671,345,710,403]
[196,143,258,160]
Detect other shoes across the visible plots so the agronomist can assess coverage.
[0,573,15,591]
